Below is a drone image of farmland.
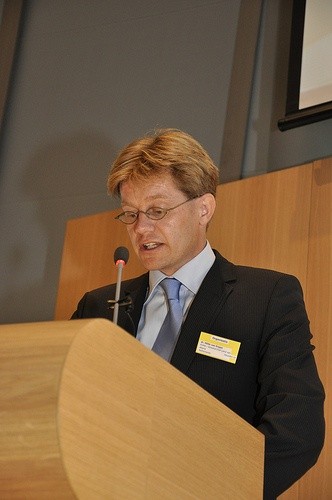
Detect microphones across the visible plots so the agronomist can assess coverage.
[112,246,129,325]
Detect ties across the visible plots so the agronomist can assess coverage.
[151,278,183,364]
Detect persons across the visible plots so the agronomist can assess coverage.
[69,128,326,500]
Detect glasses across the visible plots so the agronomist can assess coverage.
[114,192,198,225]
